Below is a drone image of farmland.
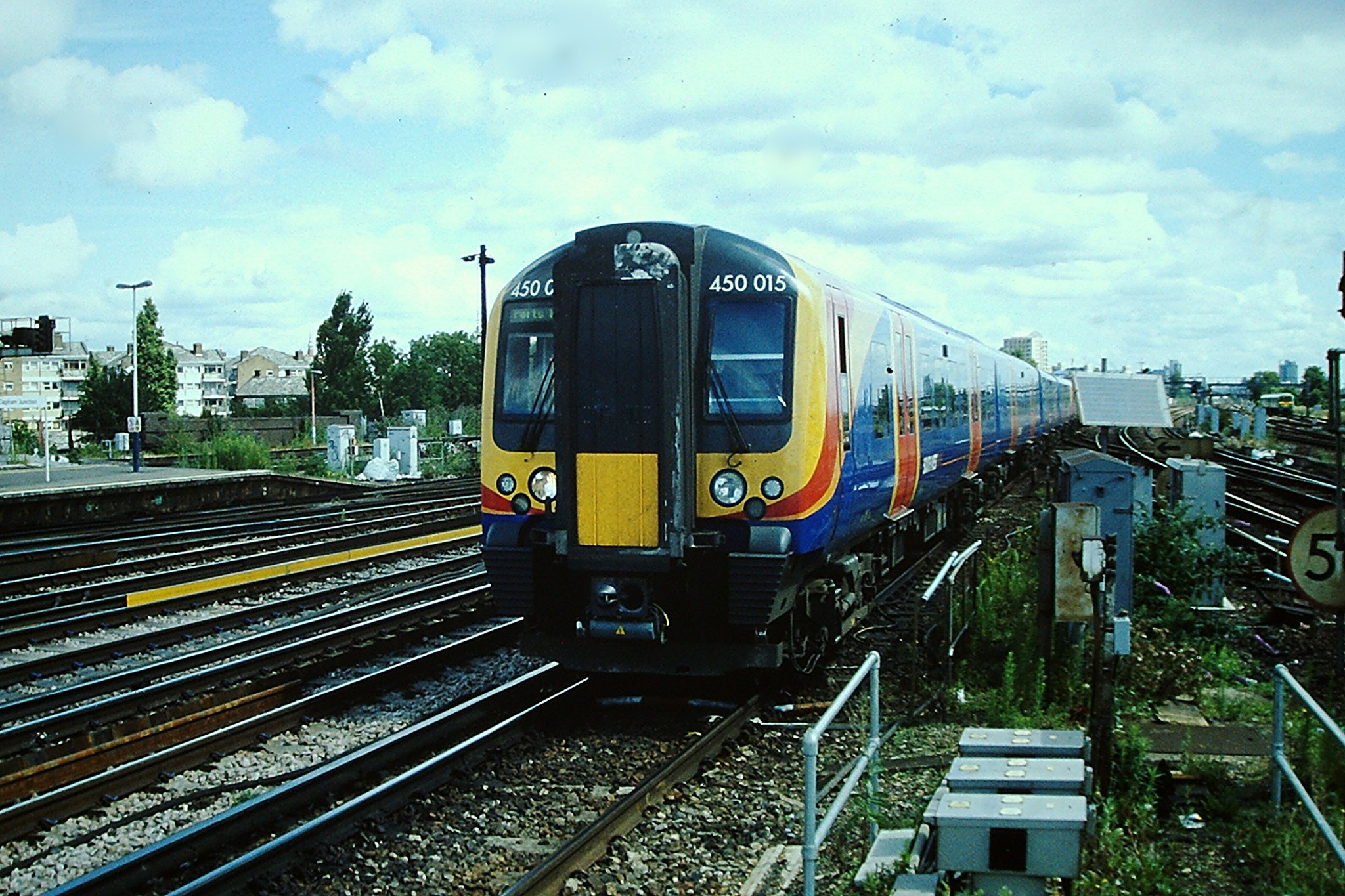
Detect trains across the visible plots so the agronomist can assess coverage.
[1260,392,1295,415]
[479,220,1080,686]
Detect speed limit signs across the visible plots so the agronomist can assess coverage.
[1289,505,1345,610]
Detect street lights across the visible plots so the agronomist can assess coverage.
[116,281,155,473]
[311,368,322,444]
[461,245,495,401]
